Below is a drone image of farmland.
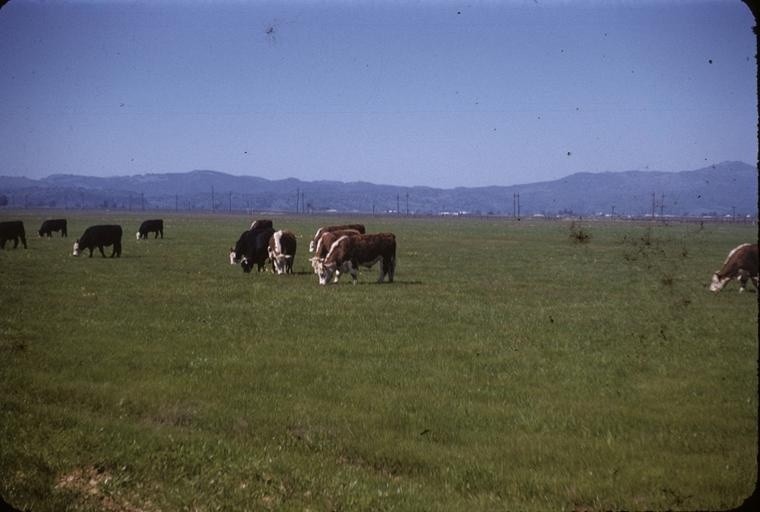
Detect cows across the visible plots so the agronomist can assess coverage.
[228,217,398,286]
[37,218,68,239]
[135,219,164,240]
[72,224,123,259]
[0,220,28,250]
[708,244,759,294]
